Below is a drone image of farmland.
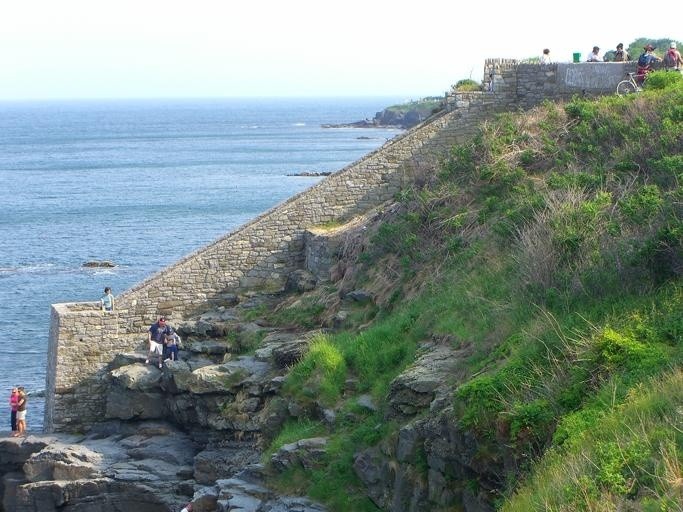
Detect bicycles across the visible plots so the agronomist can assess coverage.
[616,67,654,99]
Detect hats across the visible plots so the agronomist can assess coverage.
[158,317,165,323]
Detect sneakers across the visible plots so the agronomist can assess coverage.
[145,358,169,368]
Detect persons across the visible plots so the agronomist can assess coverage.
[615,43,633,62]
[591,47,609,62]
[164,328,182,361]
[636,44,663,87]
[145,318,169,368]
[662,42,683,72]
[539,49,552,64]
[101,287,114,311]
[10,387,19,437]
[13,386,26,437]
